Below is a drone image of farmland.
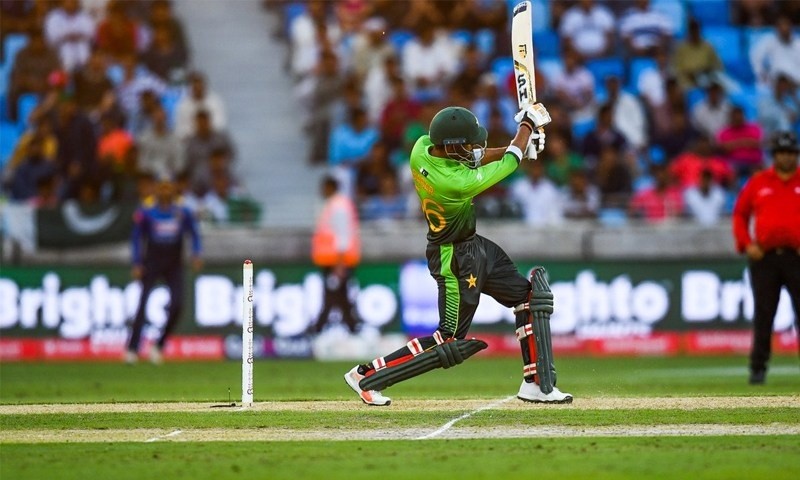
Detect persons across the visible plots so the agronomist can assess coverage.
[272,0,800,223]
[732,132,800,384]
[310,175,362,334]
[127,174,202,365]
[344,103,573,406]
[0,0,239,220]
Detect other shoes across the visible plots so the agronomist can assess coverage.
[126,349,139,364]
[750,372,765,384]
[151,347,163,363]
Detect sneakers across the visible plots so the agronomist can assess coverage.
[344,365,391,406]
[517,379,573,403]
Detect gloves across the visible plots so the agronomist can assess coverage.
[525,129,545,158]
[515,103,551,128]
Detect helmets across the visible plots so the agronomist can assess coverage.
[430,107,488,145]
[769,131,800,155]
